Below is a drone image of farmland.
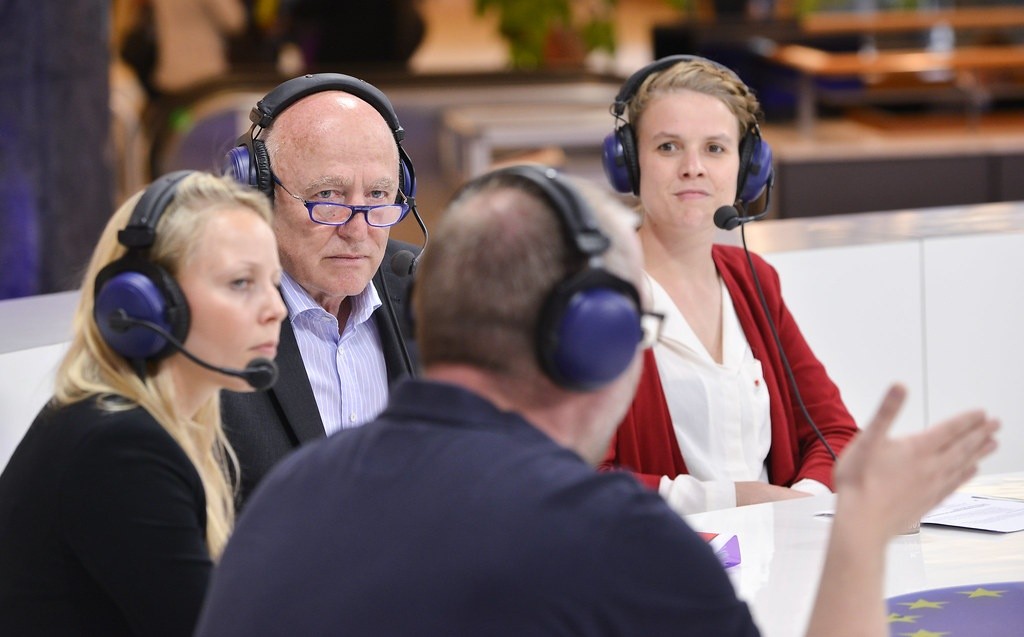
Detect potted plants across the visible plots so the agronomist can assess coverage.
[473,0,617,82]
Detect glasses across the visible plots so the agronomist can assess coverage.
[271,173,409,228]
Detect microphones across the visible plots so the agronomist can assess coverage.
[107,308,279,390]
[713,170,775,231]
[390,200,429,279]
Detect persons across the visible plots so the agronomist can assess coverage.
[589,60,860,514]
[201,160,1001,636]
[222,74,428,513]
[1,172,287,637]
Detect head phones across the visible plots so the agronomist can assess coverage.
[221,71,417,223]
[409,162,644,392]
[602,54,775,206]
[93,169,200,365]
